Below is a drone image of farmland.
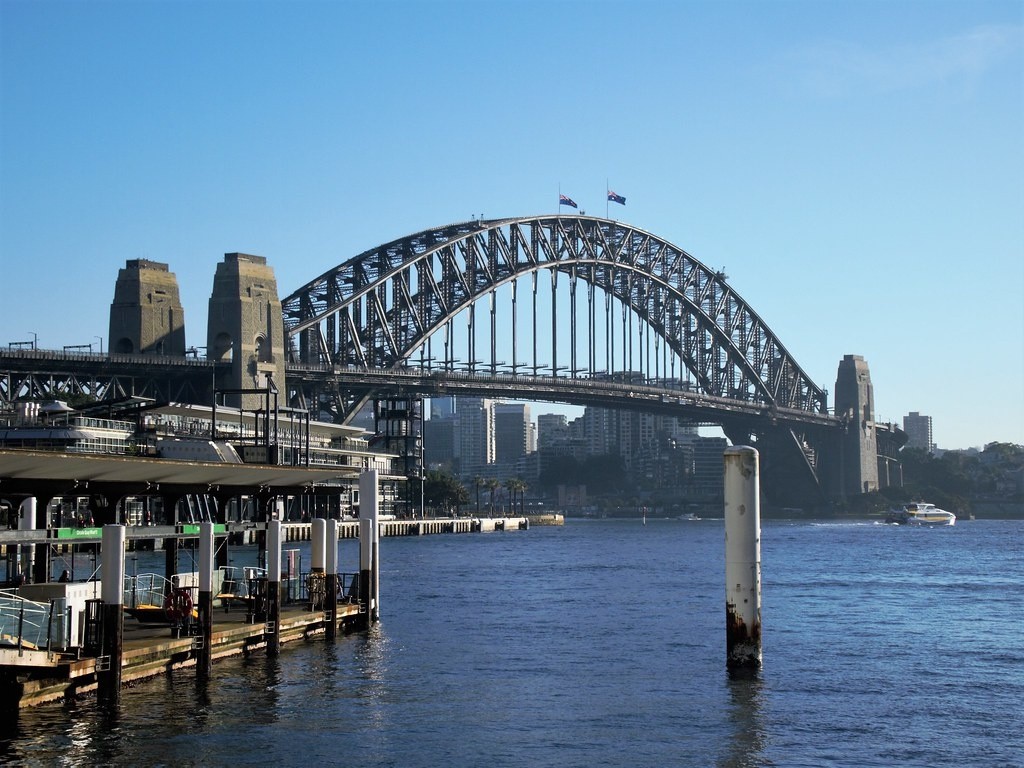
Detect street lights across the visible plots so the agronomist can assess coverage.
[162,334,167,355]
[529,503,532,513]
[27,332,37,353]
[538,502,543,514]
[96,336,102,354]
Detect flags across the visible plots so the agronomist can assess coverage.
[559,195,577,208]
[607,191,626,205]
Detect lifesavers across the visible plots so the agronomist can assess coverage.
[164,591,192,619]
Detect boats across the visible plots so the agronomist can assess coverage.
[677,513,701,521]
[886,499,956,527]
